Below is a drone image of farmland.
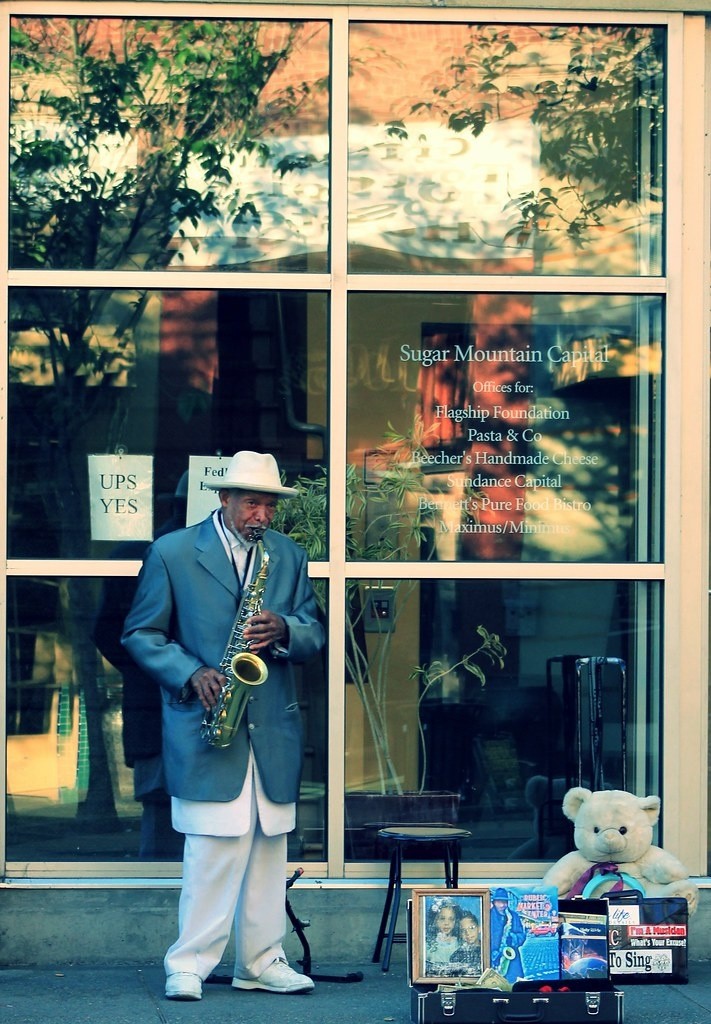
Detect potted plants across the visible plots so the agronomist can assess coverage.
[268,412,462,857]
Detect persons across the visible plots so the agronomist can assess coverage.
[425,900,459,975]
[491,889,526,985]
[121,450,328,1002]
[453,908,486,978]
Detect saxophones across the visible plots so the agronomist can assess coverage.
[197,525,273,752]
[494,907,516,977]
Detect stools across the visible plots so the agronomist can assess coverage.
[372,828,471,972]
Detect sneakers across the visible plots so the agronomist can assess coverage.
[165,972,204,1001]
[232,960,316,993]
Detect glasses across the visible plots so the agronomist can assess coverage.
[460,924,477,934]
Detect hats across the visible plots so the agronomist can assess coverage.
[492,888,512,900]
[205,450,298,499]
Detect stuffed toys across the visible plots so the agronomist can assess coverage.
[542,786,699,922]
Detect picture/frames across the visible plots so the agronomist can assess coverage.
[412,889,491,983]
